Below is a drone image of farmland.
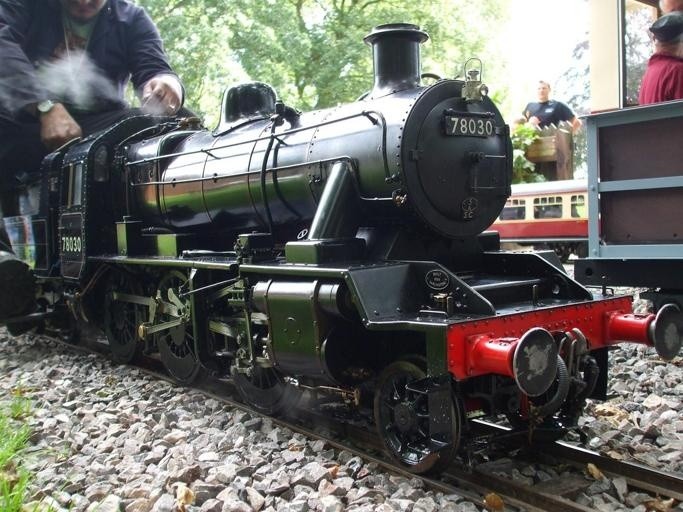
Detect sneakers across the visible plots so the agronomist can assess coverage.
[0,250,39,335]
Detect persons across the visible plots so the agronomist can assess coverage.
[636,12,683,106]
[515,80,582,181]
[0,1,204,339]
[658,1,682,16]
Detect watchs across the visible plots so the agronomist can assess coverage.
[36,98,62,118]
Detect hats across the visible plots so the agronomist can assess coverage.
[650,12,683,40]
